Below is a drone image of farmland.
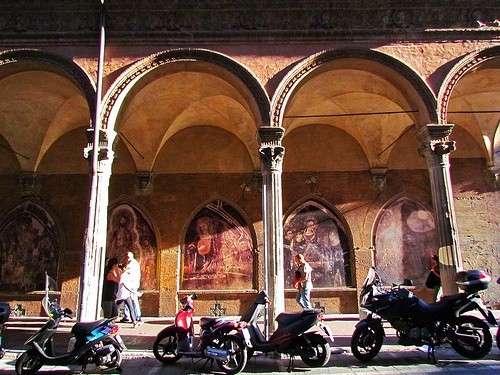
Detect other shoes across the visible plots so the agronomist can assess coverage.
[120,318,129,322]
[134,321,145,328]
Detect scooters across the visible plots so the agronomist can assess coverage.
[16,301,126,375]
[240,292,334,368]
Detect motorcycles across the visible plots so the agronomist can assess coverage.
[154,292,253,375]
[352,268,498,363]
[0,303,11,358]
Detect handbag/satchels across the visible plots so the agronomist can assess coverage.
[424,270,440,289]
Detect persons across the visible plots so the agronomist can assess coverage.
[425,254,443,303]
[294,254,313,312]
[100,251,142,328]
